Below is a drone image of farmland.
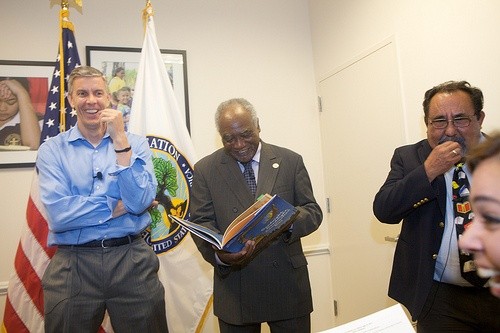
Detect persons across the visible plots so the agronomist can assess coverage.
[36,66,169,333]
[373,80,500,333]
[108,67,133,132]
[458,129,500,297]
[0,77,45,151]
[189,98,324,333]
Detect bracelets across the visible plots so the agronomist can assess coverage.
[115,145,132,153]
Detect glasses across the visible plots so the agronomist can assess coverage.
[429,116,477,129]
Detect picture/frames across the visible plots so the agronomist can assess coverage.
[85,45,190,141]
[0,60,58,168]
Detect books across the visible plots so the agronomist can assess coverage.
[168,194,301,257]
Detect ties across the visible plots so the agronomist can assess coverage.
[241,161,257,199]
[452,160,491,287]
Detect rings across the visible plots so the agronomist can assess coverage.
[452,150,458,156]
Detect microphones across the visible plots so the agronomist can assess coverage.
[452,198,464,202]
[97,172,102,180]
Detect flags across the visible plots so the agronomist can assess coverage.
[0,9,112,333]
[103,7,217,333]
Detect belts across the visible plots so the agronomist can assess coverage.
[75,232,142,247]
